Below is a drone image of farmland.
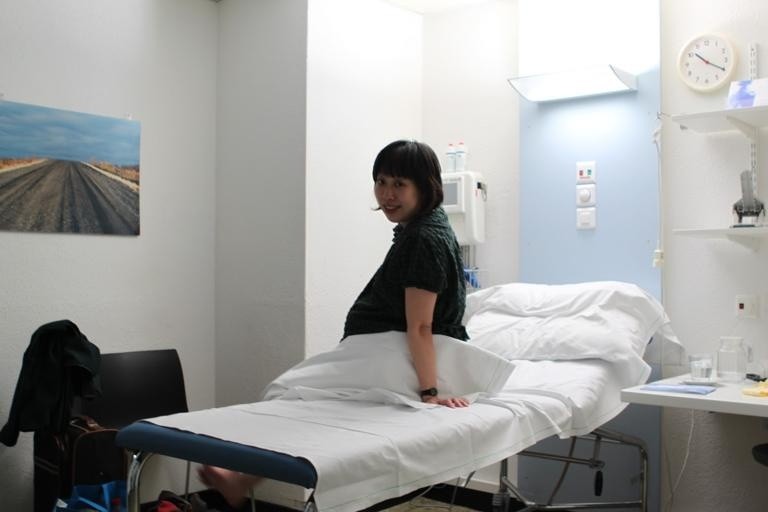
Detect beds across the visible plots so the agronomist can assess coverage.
[113,279,685,511]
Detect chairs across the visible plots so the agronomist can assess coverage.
[24,347,196,510]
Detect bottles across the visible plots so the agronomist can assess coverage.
[455,141,467,171]
[445,143,456,172]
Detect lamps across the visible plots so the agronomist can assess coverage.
[504,64,639,106]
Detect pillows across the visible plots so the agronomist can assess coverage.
[461,280,658,366]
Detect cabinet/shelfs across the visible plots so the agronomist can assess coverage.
[668,104,768,237]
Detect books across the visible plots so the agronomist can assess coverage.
[457,244,475,269]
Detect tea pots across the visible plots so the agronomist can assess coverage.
[716,334,754,382]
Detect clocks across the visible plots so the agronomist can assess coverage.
[676,33,738,94]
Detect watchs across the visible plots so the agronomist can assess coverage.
[419,387,438,396]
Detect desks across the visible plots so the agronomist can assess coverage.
[618,370,767,421]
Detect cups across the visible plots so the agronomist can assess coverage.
[687,353,714,384]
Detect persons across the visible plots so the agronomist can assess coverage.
[195,140,470,507]
